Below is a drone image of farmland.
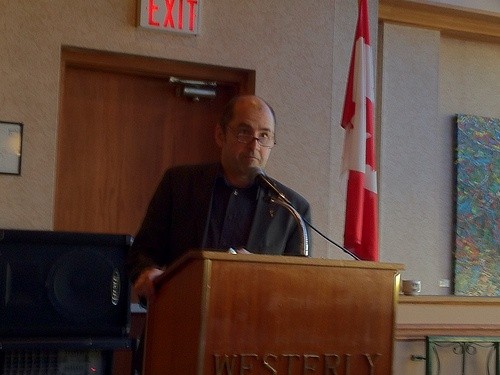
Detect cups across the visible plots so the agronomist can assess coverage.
[402,280,421,296]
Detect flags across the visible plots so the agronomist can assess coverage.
[339,0,380,262]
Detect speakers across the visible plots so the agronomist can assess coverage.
[0,229,137,348]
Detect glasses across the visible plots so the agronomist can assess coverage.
[226,127,275,150]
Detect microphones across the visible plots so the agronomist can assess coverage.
[248,167,293,205]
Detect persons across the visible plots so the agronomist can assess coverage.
[126,94,311,374]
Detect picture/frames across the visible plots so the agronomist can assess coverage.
[0,120,24,175]
